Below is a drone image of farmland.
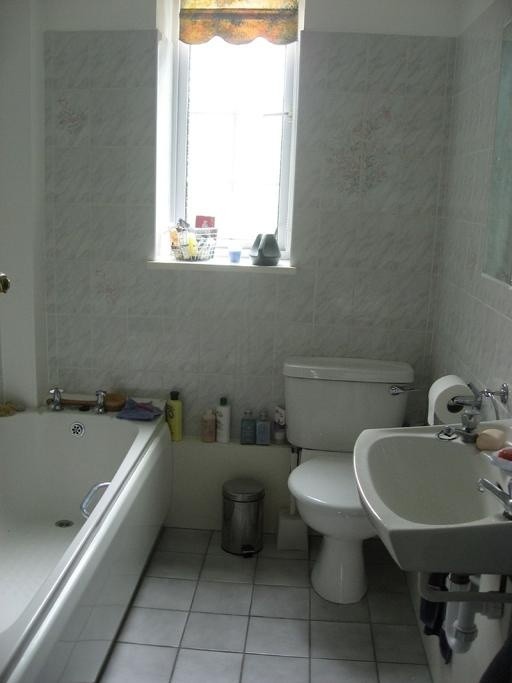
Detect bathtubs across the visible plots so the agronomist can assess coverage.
[0,392,175,682]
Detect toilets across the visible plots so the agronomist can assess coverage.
[283,355,416,605]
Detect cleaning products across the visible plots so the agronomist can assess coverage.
[165,390,182,442]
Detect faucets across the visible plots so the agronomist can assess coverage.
[92,388,106,415]
[475,470,512,517]
[48,384,62,411]
[444,408,478,442]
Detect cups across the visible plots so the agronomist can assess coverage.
[228,239,241,262]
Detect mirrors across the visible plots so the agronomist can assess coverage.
[478,20,512,289]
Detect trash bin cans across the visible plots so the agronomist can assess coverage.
[221,477,265,558]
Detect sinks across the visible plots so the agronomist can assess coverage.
[351,418,512,576]
